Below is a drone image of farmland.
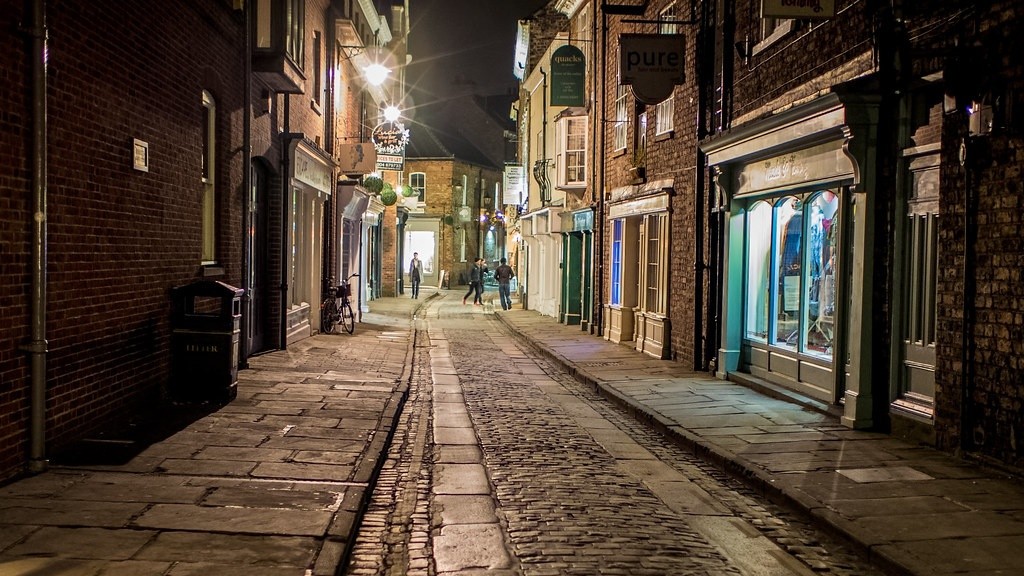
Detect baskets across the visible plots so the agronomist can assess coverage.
[336,284,352,297]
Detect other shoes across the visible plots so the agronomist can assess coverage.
[503,305,507,310]
[411,295,414,298]
[463,298,466,305]
[415,295,417,299]
[508,303,511,310]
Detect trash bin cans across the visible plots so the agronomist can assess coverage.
[166,276,245,403]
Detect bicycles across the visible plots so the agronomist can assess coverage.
[320,272,360,334]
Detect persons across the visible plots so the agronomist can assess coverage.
[493,258,514,310]
[462,257,480,306]
[473,258,488,305]
[409,252,424,299]
[779,197,825,323]
